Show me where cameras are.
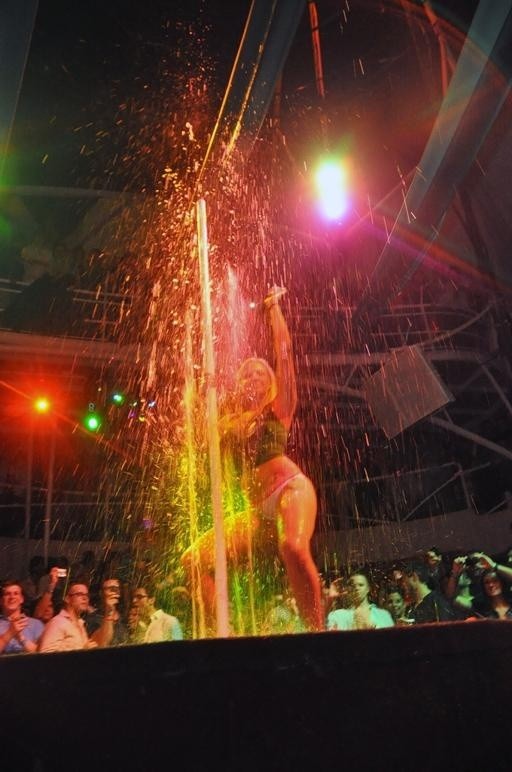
[57,569,67,577]
[335,579,355,592]
[461,556,477,567]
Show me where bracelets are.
[268,302,279,311]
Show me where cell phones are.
[110,585,120,592]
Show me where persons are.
[2,545,511,656]
[180,285,327,639]
